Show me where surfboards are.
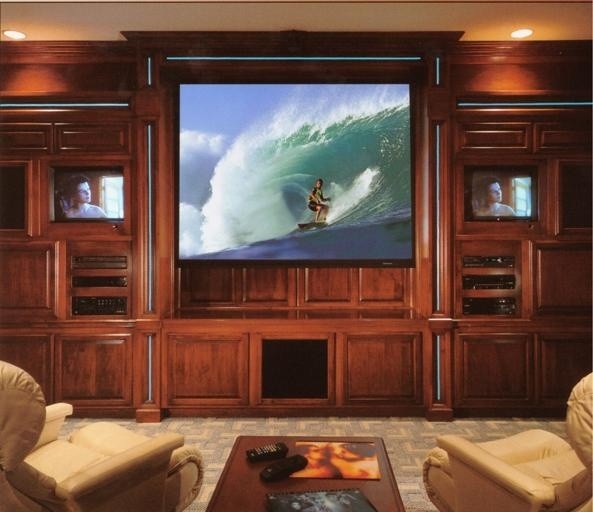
[297,221,328,227]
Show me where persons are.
[308,179,330,222]
[293,444,342,478]
[472,176,516,216]
[290,499,301,511]
[64,175,107,217]
[328,442,379,479]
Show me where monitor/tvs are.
[54,165,126,222]
[464,164,538,222]
[173,78,417,271]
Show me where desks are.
[205,435,406,512]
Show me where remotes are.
[246,442,288,462]
[260,455,308,482]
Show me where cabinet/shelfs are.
[161,319,437,419]
[2,39,145,321]
[454,40,593,319]
[1,327,150,418]
[440,325,593,419]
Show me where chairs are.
[423,373,593,512]
[0,360,205,512]
